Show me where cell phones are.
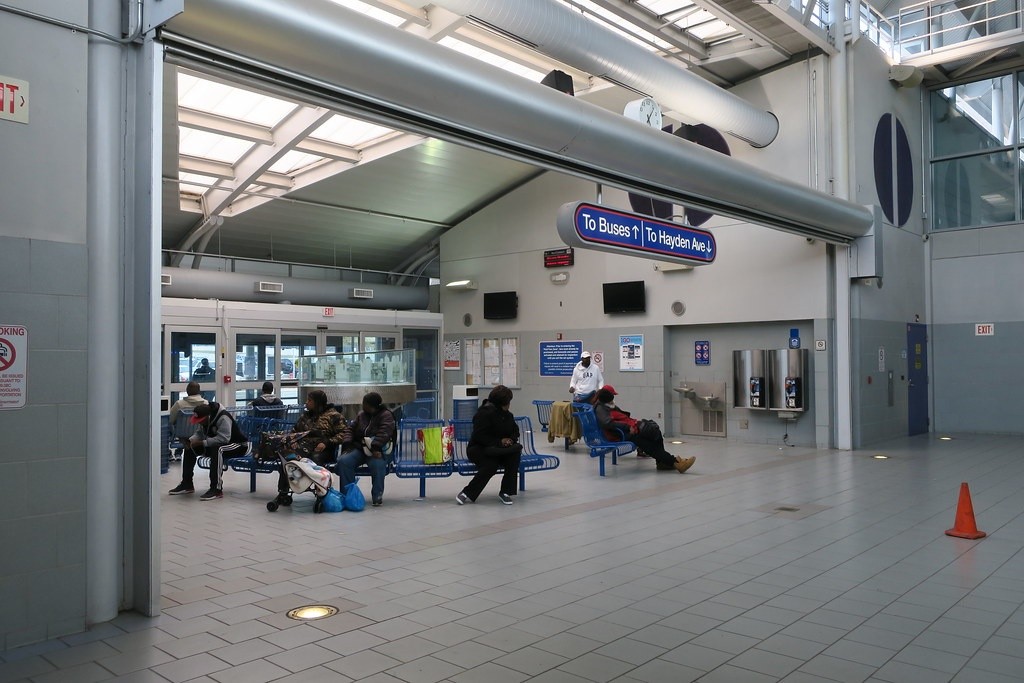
[177,435,189,442]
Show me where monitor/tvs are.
[484,292,517,319]
[602,280,646,313]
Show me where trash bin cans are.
[453,384,478,441]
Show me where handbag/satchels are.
[322,487,345,512]
[417,426,453,464]
[362,437,393,456]
[344,477,365,511]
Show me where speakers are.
[540,69,574,96]
[672,126,702,145]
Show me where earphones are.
[209,416,210,419]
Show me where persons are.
[169,382,210,461]
[456,385,523,505]
[592,385,696,474]
[247,381,283,437]
[569,351,604,445]
[273,390,348,505]
[169,401,248,501]
[190,359,215,403]
[337,392,395,507]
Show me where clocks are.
[624,96,662,131]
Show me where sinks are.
[699,396,720,401]
[672,388,694,392]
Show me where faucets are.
[684,383,688,389]
[710,392,714,397]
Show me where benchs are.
[170,397,635,498]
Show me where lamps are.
[658,260,693,272]
[446,279,478,290]
[888,65,925,90]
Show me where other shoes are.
[200,487,223,500]
[372,492,382,505]
[674,455,696,473]
[273,494,286,503]
[169,480,194,494]
[499,491,513,504]
[636,451,653,459]
[456,491,467,504]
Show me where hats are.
[191,404,211,423]
[581,351,591,358]
[602,385,618,395]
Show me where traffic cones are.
[945,482,986,539]
